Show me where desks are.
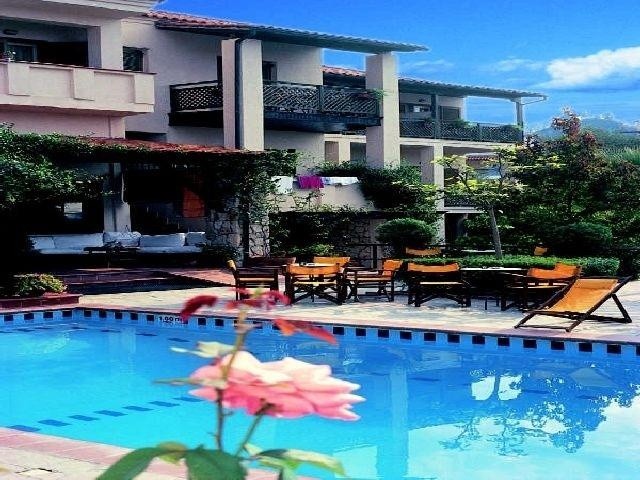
[82,246,140,267]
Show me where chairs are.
[227,252,403,306]
[404,247,582,311]
[514,275,632,332]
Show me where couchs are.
[27,232,206,255]
